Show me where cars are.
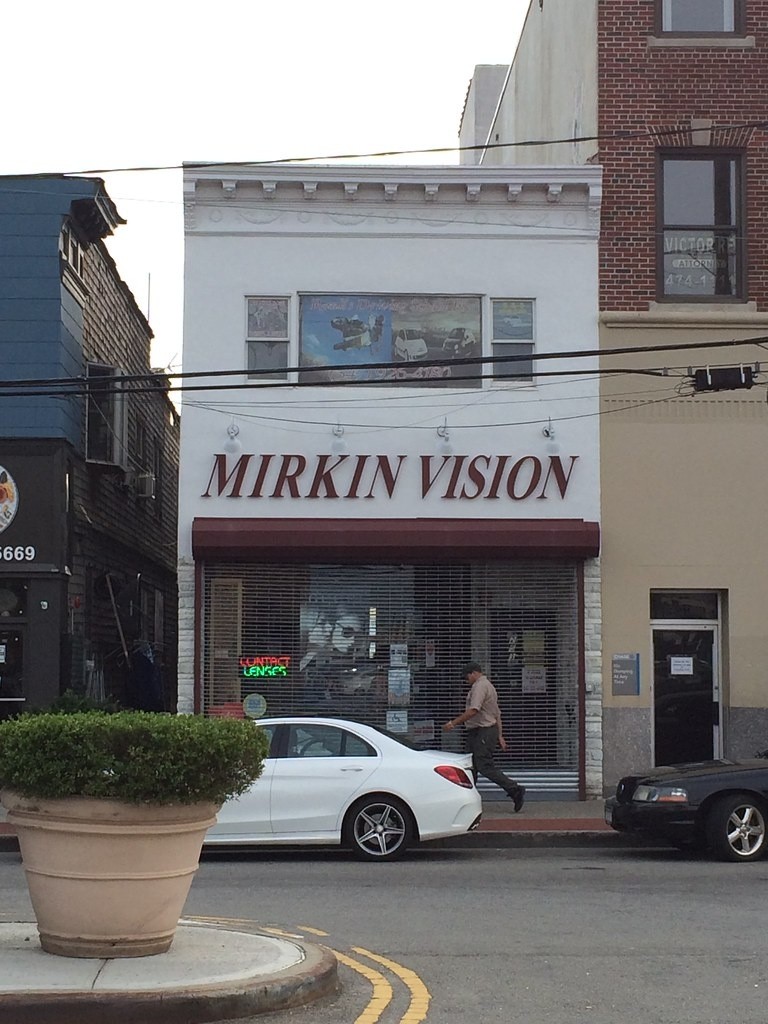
[604,751,768,861]
[200,718,482,861]
[337,664,421,698]
[656,690,718,749]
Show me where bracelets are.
[448,720,455,730]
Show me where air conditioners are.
[135,474,156,497]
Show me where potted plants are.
[0,711,269,958]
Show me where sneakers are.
[506,785,526,812]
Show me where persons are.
[442,661,525,812]
